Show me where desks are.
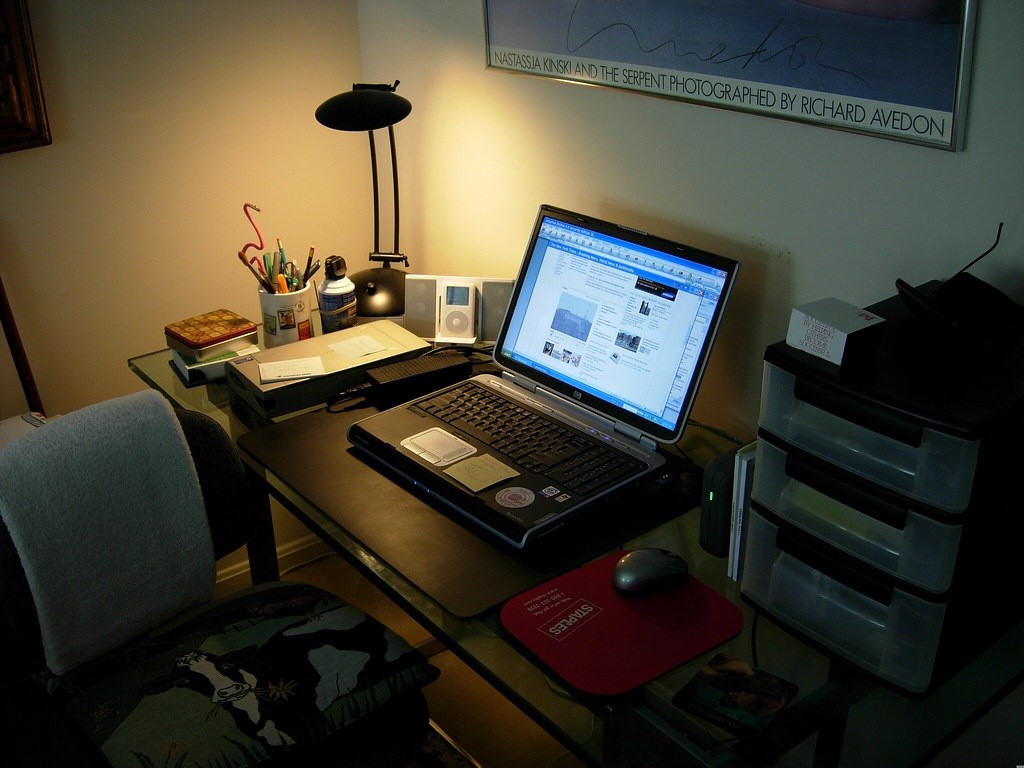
[128,323,1024,768]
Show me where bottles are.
[317,255,357,334]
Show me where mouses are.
[612,547,689,598]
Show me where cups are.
[258,281,314,349]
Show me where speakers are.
[402,272,516,342]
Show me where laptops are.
[345,204,741,551]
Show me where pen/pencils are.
[238,201,323,295]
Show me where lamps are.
[315,80,412,316]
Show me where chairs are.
[0,389,440,768]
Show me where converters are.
[345,349,474,411]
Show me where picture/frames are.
[0,0,52,155]
